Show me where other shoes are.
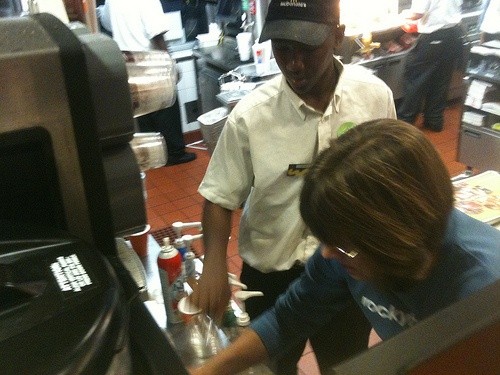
[421,122,443,132]
[167,152,196,164]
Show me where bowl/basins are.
[196,33,220,47]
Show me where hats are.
[258,0,340,46]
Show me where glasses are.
[334,246,360,264]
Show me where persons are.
[188,0,398,375]
[396,0,464,133]
[188,117,500,375]
[99,0,197,167]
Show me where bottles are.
[157,237,185,324]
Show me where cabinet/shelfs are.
[457,38,500,173]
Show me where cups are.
[128,224,151,257]
[177,296,203,325]
[121,49,176,170]
[236,32,252,61]
